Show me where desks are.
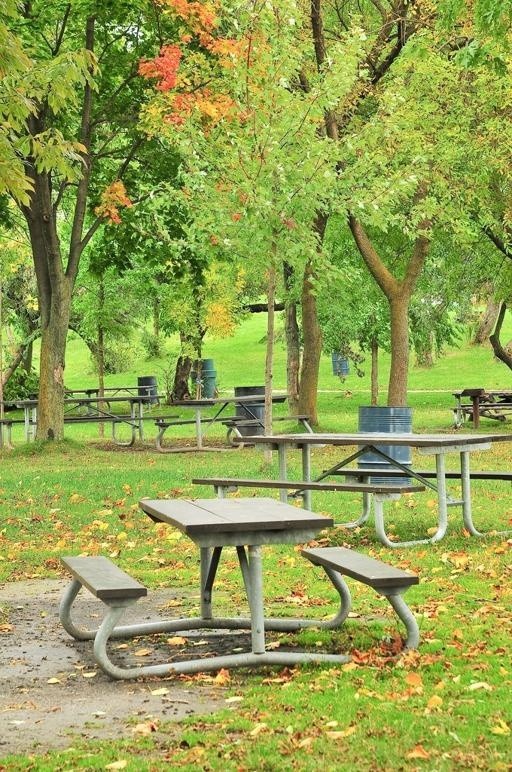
[138,498,335,654]
[1,385,288,452]
[235,433,511,547]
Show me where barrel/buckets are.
[137,376,160,405]
[331,351,351,376]
[191,370,217,399]
[233,386,265,438]
[358,405,413,486]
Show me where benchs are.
[193,469,512,547]
[222,415,315,452]
[298,546,420,668]
[53,555,147,681]
